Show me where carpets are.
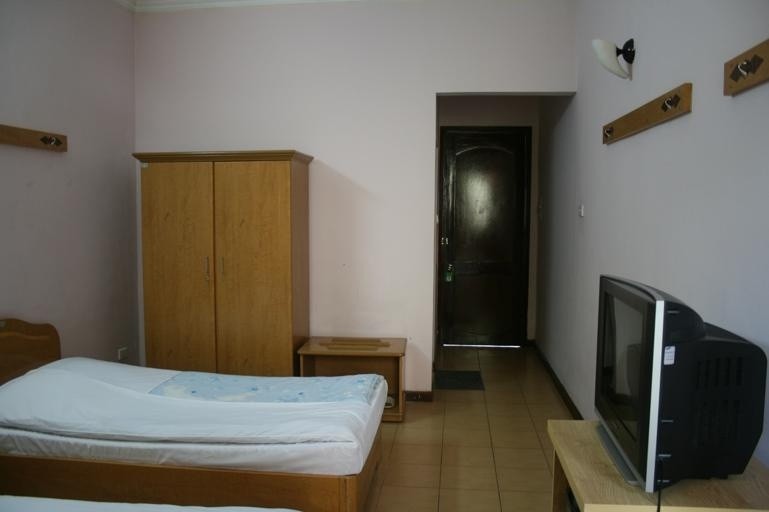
[432,368,485,392]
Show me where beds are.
[2,316,390,511]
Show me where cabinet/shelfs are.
[297,334,408,426]
[132,147,313,375]
[545,419,769,512]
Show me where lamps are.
[589,36,636,79]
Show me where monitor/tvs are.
[593,274,767,496]
